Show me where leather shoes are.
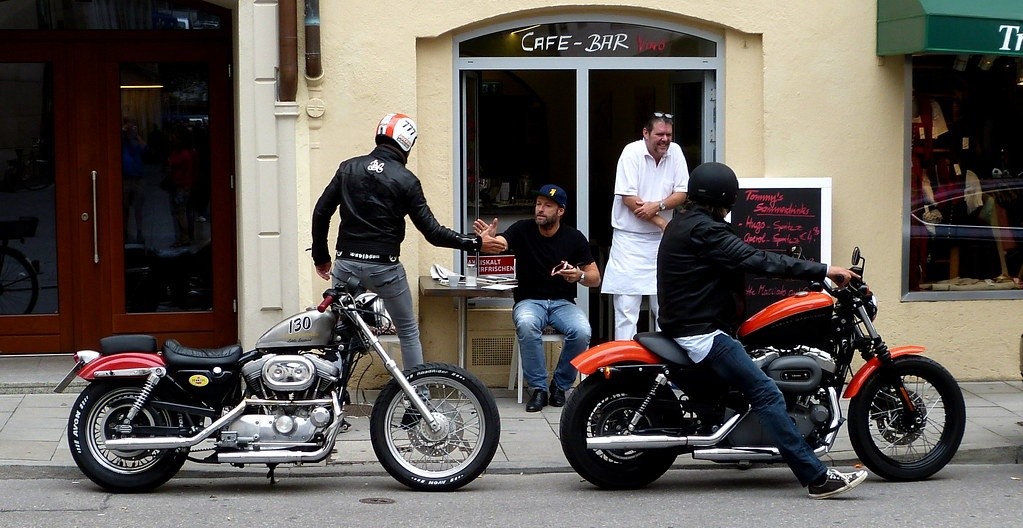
[526,389,548,412]
[548,379,566,407]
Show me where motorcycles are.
[54,272,501,495]
[559,244,966,490]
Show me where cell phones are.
[551,262,568,276]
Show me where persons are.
[656,162,868,500]
[311,113,505,432]
[473,184,601,412]
[122,117,148,244]
[600,112,690,341]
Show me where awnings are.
[876,0,1023,59]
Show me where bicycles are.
[0,218,44,316]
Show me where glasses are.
[647,112,673,126]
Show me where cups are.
[448,273,459,287]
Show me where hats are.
[530,184,566,208]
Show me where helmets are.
[375,114,418,151]
[688,162,738,210]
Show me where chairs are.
[510,258,589,404]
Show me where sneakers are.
[807,470,867,499]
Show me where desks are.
[418,275,516,402]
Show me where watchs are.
[659,201,665,211]
[579,273,585,283]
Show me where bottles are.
[465,264,478,287]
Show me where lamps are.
[977,55,998,71]
[1015,57,1023,86]
[952,53,972,71]
